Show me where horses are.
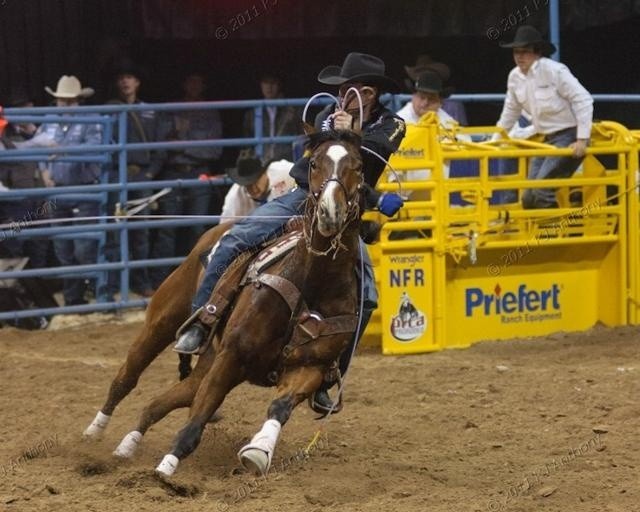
[81,128,364,482]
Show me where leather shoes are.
[174,320,211,357]
[310,388,337,412]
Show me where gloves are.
[377,190,404,219]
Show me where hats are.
[404,73,458,96]
[317,52,401,89]
[45,73,96,98]
[405,56,454,70]
[224,149,277,187]
[497,27,558,56]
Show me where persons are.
[220,154,296,224]
[0,103,66,266]
[387,73,459,183]
[36,75,105,314]
[487,24,593,209]
[174,50,407,413]
[230,66,303,161]
[106,60,157,307]
[404,55,467,126]
[152,61,223,288]
